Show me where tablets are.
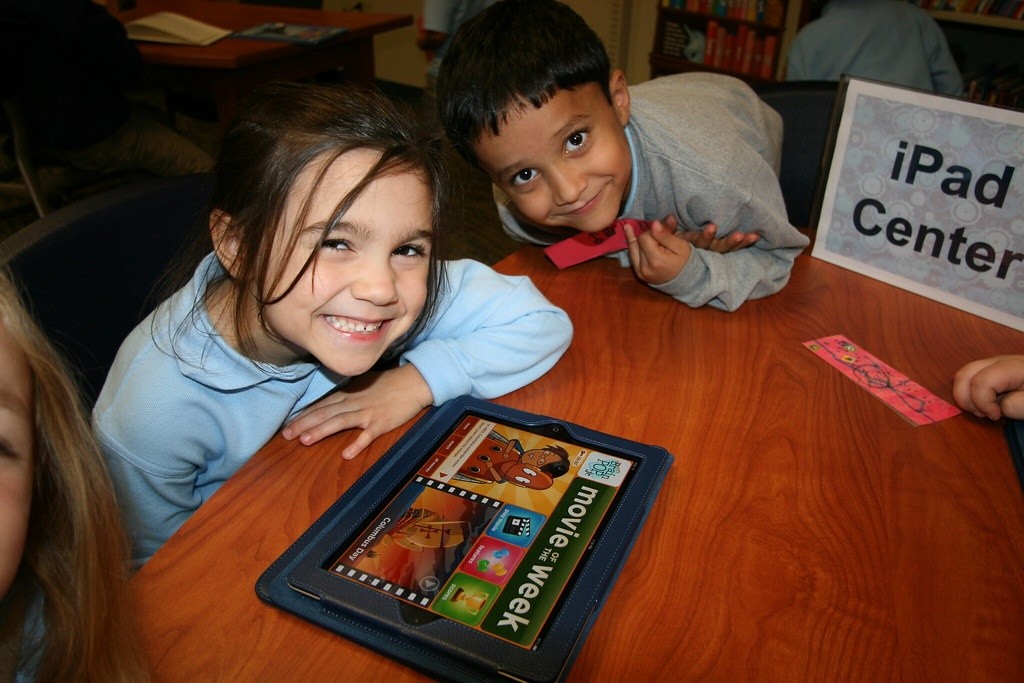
[256,395,676,683]
[227,20,347,47]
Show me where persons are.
[1,0,222,197]
[951,351,1024,418]
[0,271,149,683]
[436,0,809,314]
[779,0,963,104]
[89,63,575,569]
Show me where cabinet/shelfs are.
[649,0,790,99]
[909,0,1024,113]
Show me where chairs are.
[0,93,218,264]
[748,76,849,220]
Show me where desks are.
[126,244,1024,683]
[109,0,414,232]
